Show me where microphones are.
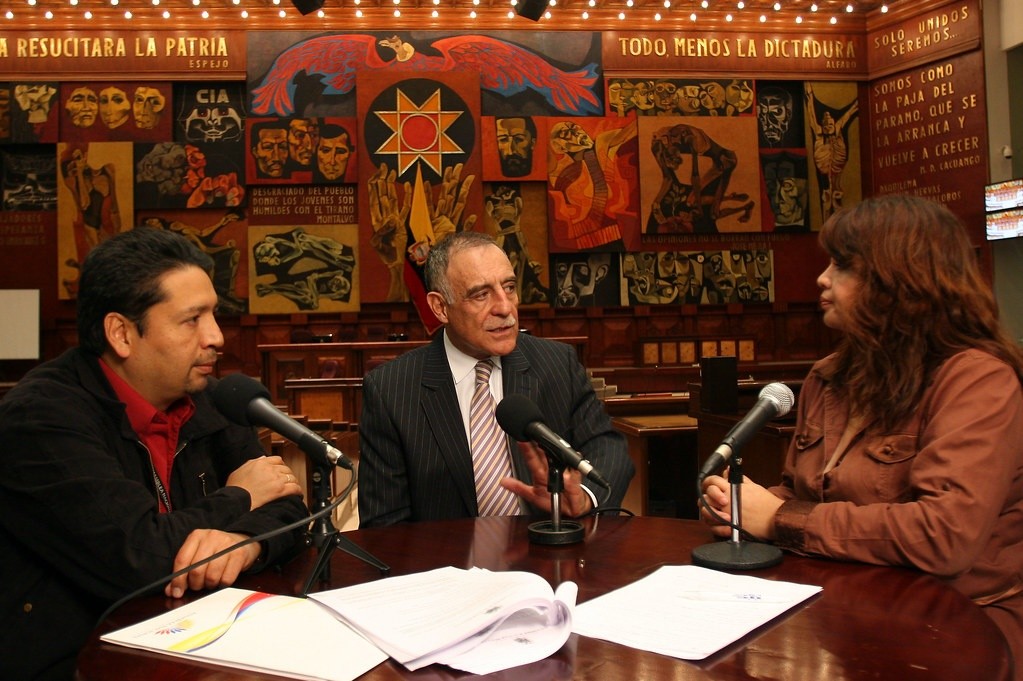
[697,383,795,480]
[495,394,610,490]
[213,373,354,472]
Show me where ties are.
[471,358,522,518]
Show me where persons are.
[693,190,1021,678]
[0,227,316,681]
[353,234,638,530]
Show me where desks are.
[598,379,798,517]
[75,517,1013,681]
[257,335,588,453]
